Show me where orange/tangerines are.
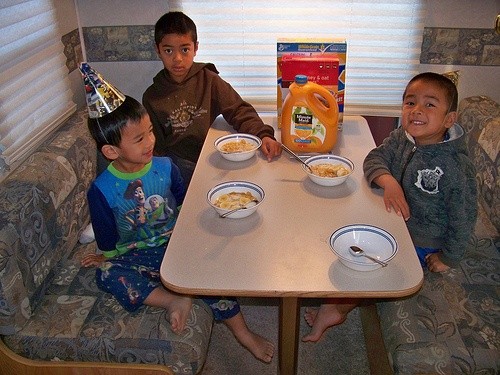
[290,128,322,148]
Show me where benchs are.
[375,96,500,375]
[0,106,212,375]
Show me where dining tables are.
[160,112,424,375]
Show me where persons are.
[81,95,275,363]
[78,12,282,245]
[302,72,479,342]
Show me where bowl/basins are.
[214,133,262,162]
[205,180,266,218]
[327,223,399,272]
[302,154,354,187]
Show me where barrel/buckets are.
[281,74,338,154]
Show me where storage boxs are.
[276,38,346,131]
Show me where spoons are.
[348,246,388,267]
[220,200,259,218]
